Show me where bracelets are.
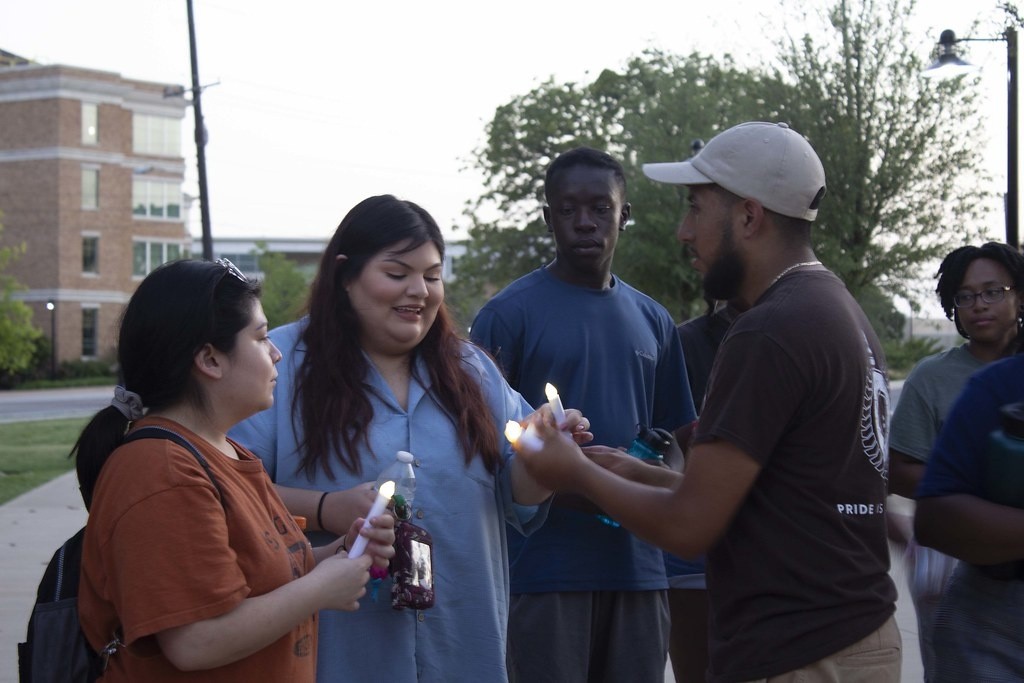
[317,491,329,532]
[334,534,347,552]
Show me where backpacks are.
[18,427,224,683]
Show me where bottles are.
[373,450,418,527]
[595,422,674,528]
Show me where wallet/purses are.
[391,521,435,612]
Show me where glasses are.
[953,286,1013,307]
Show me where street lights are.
[46,299,56,381]
[920,26,1018,247]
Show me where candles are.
[545,382,574,441]
[348,480,396,559]
[504,421,544,453]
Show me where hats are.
[642,120,826,222]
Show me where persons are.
[225,192,593,682]
[668,296,749,682]
[503,120,903,682]
[912,349,1022,682]
[466,148,696,683]
[889,241,1022,682]
[66,256,395,682]
